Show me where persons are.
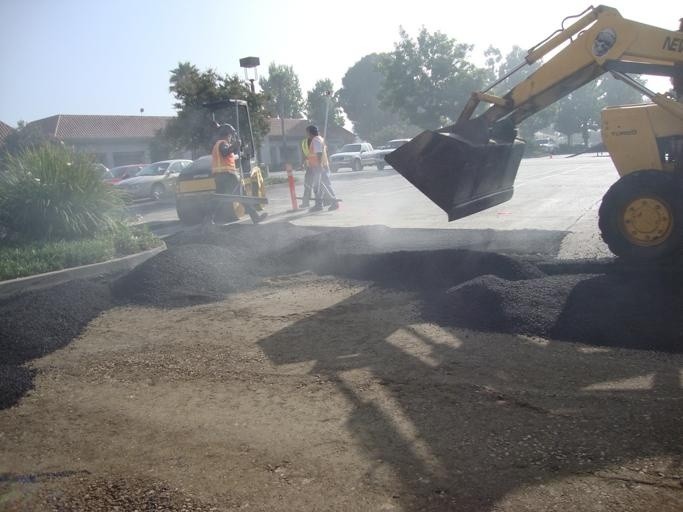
[210,123,269,223]
[305,126,340,212]
[299,136,331,207]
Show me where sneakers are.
[299,203,339,212]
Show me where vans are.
[376,138,412,170]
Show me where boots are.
[250,212,268,223]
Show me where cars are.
[175,151,267,221]
[90,159,193,204]
[535,137,559,154]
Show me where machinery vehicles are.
[383,6,682,263]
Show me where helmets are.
[217,123,235,135]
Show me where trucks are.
[328,142,398,171]
